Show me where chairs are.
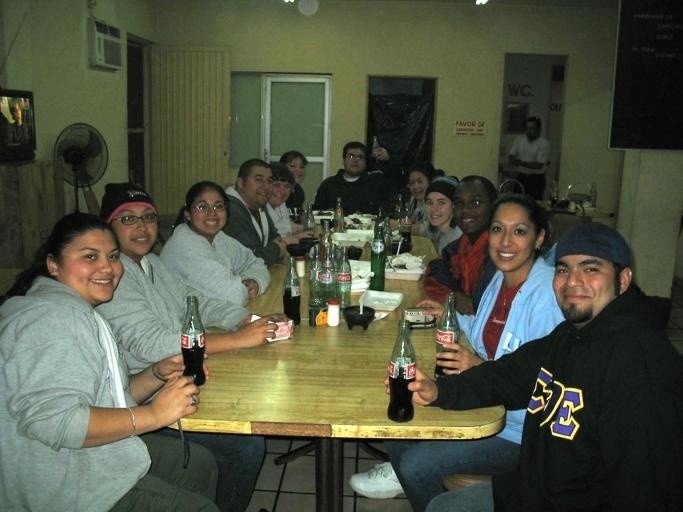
[549,212,592,247]
[499,177,526,198]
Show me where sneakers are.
[349,462,407,499]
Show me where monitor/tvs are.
[0,89,36,163]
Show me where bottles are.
[434,294,460,379]
[387,320,417,423]
[180,295,206,387]
[283,194,413,327]
[372,136,379,156]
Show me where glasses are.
[345,153,362,161]
[190,202,228,212]
[112,212,157,225]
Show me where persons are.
[389,163,462,253]
[510,118,550,201]
[348,176,503,498]
[389,197,570,511]
[96,182,295,512]
[312,141,407,214]
[223,151,307,268]
[385,223,683,510]
[161,181,271,308]
[0,212,220,512]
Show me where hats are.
[423,176,459,205]
[554,222,631,266]
[99,182,159,225]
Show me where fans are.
[52,121,110,218]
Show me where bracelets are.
[151,359,167,385]
[127,406,139,439]
